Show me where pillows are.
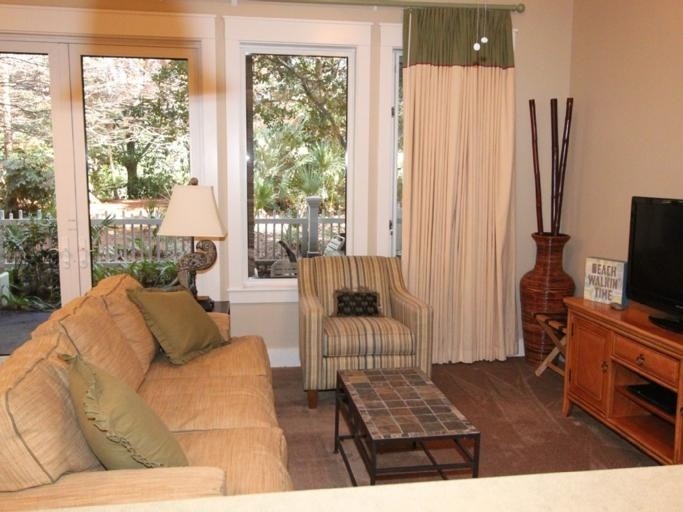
[329,284,386,320]
[122,284,225,366]
[54,353,190,472]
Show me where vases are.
[515,232,575,368]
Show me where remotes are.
[611,302,624,310]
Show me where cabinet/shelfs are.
[560,293,682,466]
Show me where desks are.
[198,300,230,315]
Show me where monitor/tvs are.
[627,197,683,335]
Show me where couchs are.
[289,255,434,410]
[0,270,297,510]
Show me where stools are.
[528,310,569,379]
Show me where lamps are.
[155,175,227,313]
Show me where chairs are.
[249,234,344,275]
